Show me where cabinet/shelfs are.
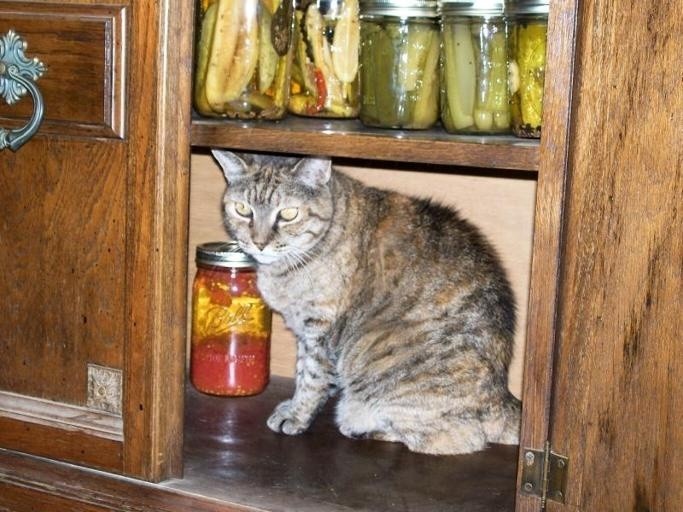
[515,4,683,512]
[0,0,157,510]
[158,1,581,512]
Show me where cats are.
[209,146,522,458]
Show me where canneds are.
[193,0,285,122]
[502,0,550,139]
[440,0,510,136]
[285,0,360,120]
[188,241,272,399]
[357,1,439,132]
